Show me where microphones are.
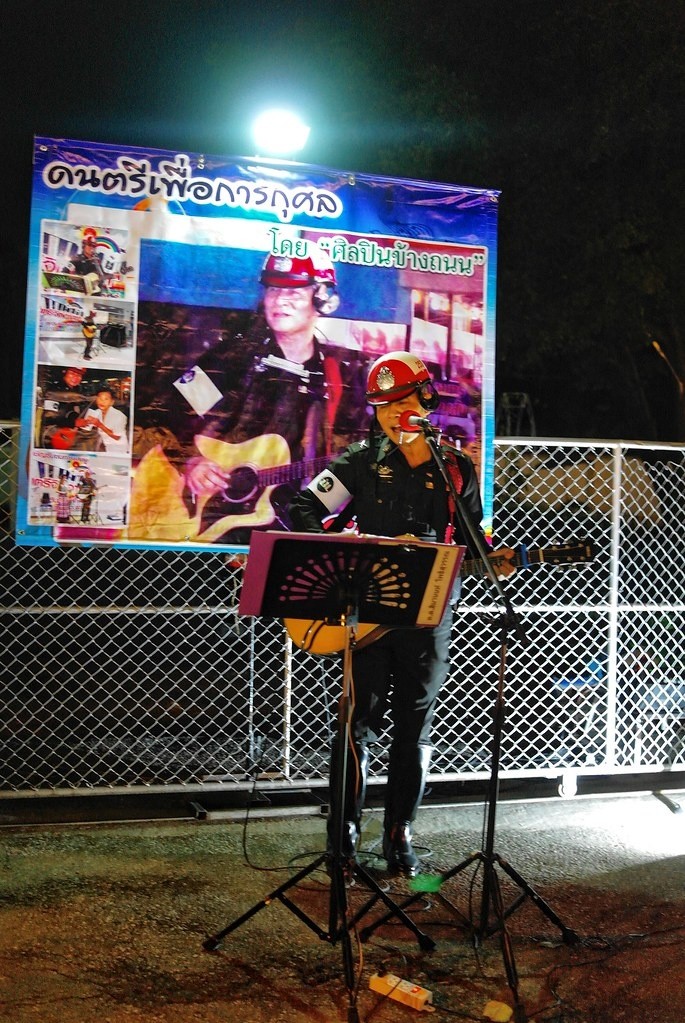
[398,410,432,432]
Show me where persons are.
[138,238,379,546]
[59,235,116,296]
[81,309,102,360]
[285,351,513,889]
[39,366,129,452]
[56,471,98,522]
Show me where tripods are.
[68,489,105,527]
[81,329,110,359]
[357,427,576,1016]
[201,538,438,992]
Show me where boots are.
[383,745,431,877]
[328,736,370,851]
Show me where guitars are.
[284,534,595,657]
[130,434,341,543]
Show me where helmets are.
[258,237,338,288]
[68,367,87,375]
[83,234,97,247]
[366,351,430,405]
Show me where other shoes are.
[84,354,93,361]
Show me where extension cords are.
[369,971,436,1012]
